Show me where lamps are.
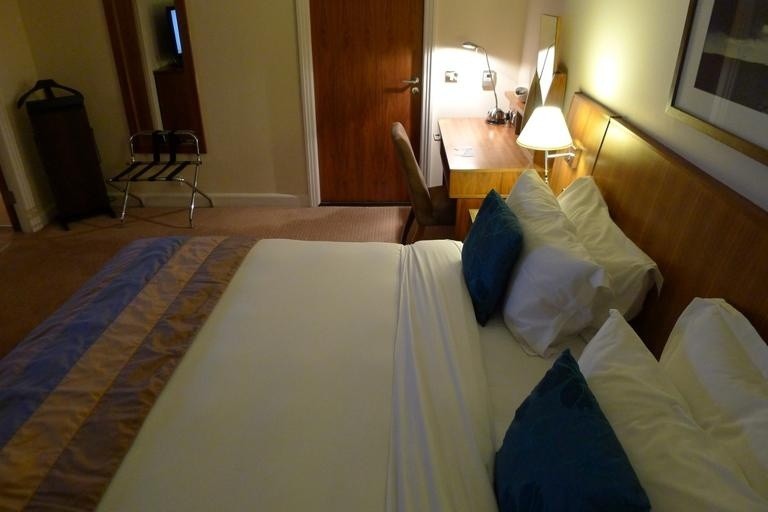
[461,41,504,119]
[516,106,583,184]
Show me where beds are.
[2,93,768,512]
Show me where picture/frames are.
[664,0,767,160]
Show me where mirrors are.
[536,13,560,109]
[102,0,208,155]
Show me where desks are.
[437,114,547,241]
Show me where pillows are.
[494,347,653,511]
[462,189,523,325]
[556,174,664,344]
[578,308,767,511]
[660,296,768,495]
[503,167,610,358]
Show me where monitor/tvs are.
[167,6,183,58]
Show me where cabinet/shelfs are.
[152,64,204,146]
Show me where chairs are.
[106,130,214,228]
[391,121,457,245]
